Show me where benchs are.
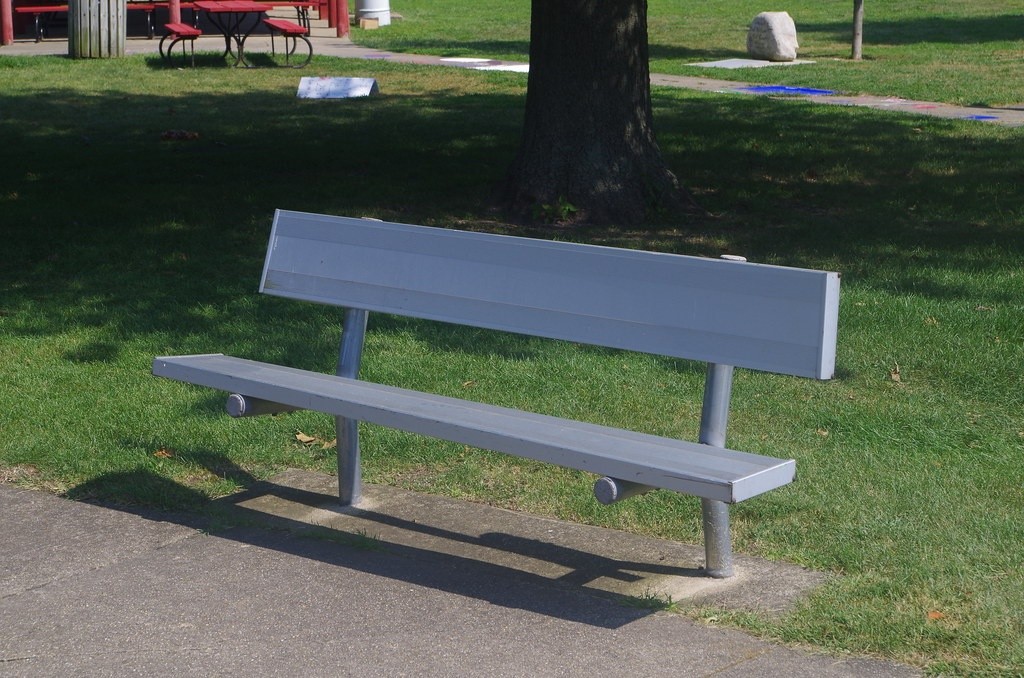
[262,19,314,69]
[157,0,320,38]
[159,23,203,71]
[154,209,842,584]
[14,3,154,44]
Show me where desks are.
[193,1,273,70]
